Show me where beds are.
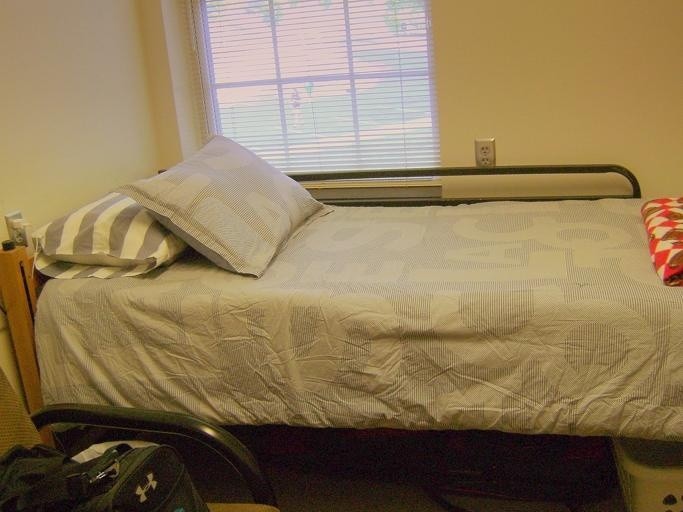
[0,162,683,453]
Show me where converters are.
[13,218,25,230]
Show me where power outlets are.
[4,210,29,248]
[473,136,497,168]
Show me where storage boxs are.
[611,437,683,512]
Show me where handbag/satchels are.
[14,440,210,512]
